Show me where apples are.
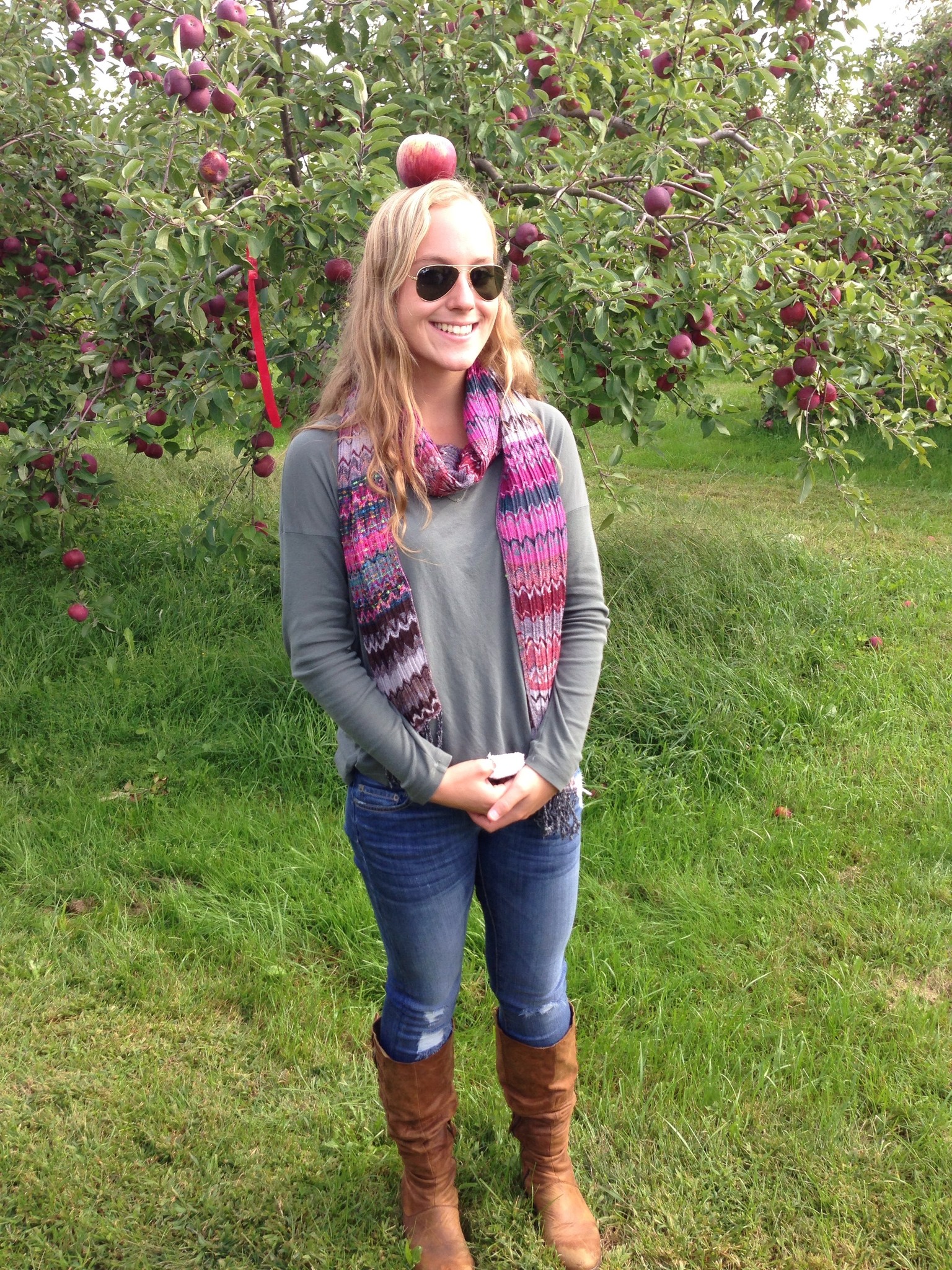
[900,595,918,612]
[587,780,607,798]
[62,597,92,619]
[772,804,792,822]
[1,1,952,572]
[863,632,883,653]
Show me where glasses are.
[407,264,505,302]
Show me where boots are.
[494,1000,601,1270]
[370,1013,475,1270]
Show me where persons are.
[281,177,609,1269]
